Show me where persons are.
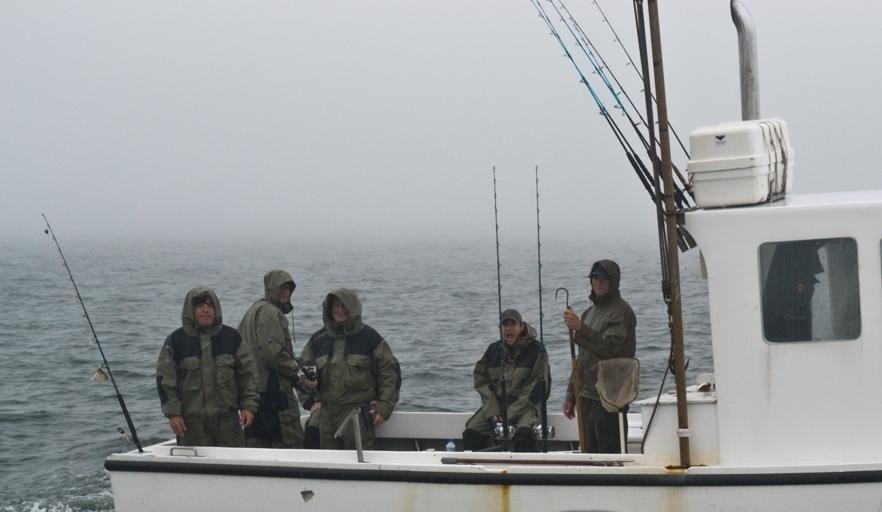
[295,287,401,449]
[462,308,551,451]
[236,269,306,449]
[155,286,261,447]
[562,260,639,453]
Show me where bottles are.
[446,438,456,450]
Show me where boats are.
[40,0,882,512]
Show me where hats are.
[588,266,610,278]
[500,309,520,323]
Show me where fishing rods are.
[492,165,509,452]
[533,1,697,253]
[40,212,144,453]
[267,334,318,381]
[535,166,549,451]
